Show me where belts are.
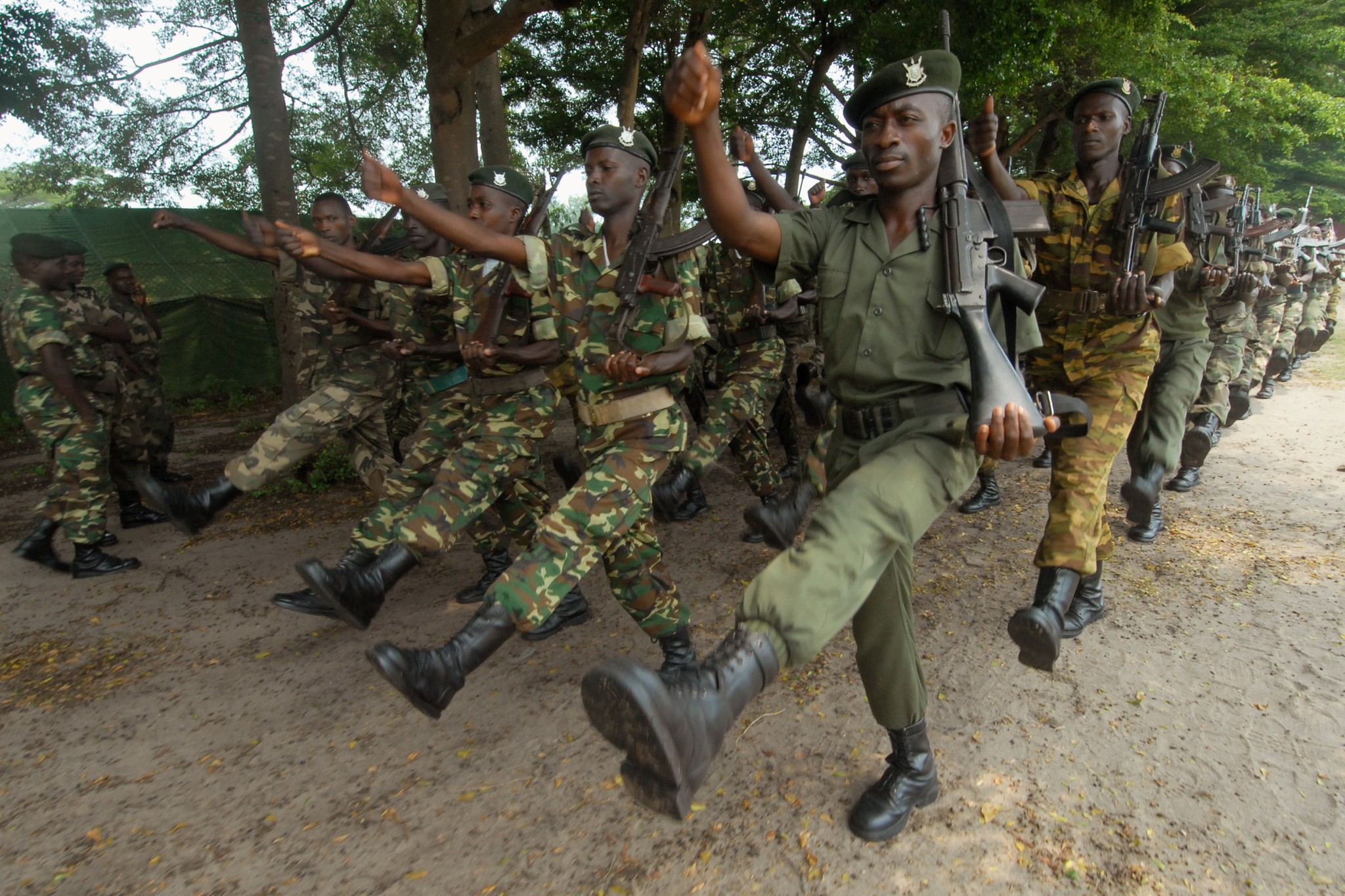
[1039,288,1114,314]
[319,316,971,440]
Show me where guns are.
[611,147,718,366]
[1185,140,1238,273]
[737,272,769,318]
[327,204,414,306]
[1330,237,1345,278]
[1232,182,1284,273]
[1253,186,1292,268]
[1109,92,1221,300]
[1314,238,1329,278]
[471,168,567,346]
[1005,155,1014,176]
[1287,186,1316,282]
[917,8,1051,436]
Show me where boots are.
[364,602,517,718]
[456,548,514,603]
[521,583,591,641]
[15,466,244,577]
[581,630,779,823]
[848,721,939,839]
[655,631,698,673]
[271,543,378,620]
[549,363,834,551]
[958,317,1337,674]
[296,545,419,630]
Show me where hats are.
[60,238,87,255]
[843,50,961,132]
[102,262,129,275]
[580,124,657,172]
[1064,78,1141,121]
[1153,144,1332,233]
[841,152,868,170]
[410,183,448,203]
[741,180,766,205]
[10,233,63,258]
[468,165,534,209]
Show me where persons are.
[151,165,590,644]
[577,78,1342,672]
[0,232,195,581]
[357,125,699,723]
[580,40,1061,842]
